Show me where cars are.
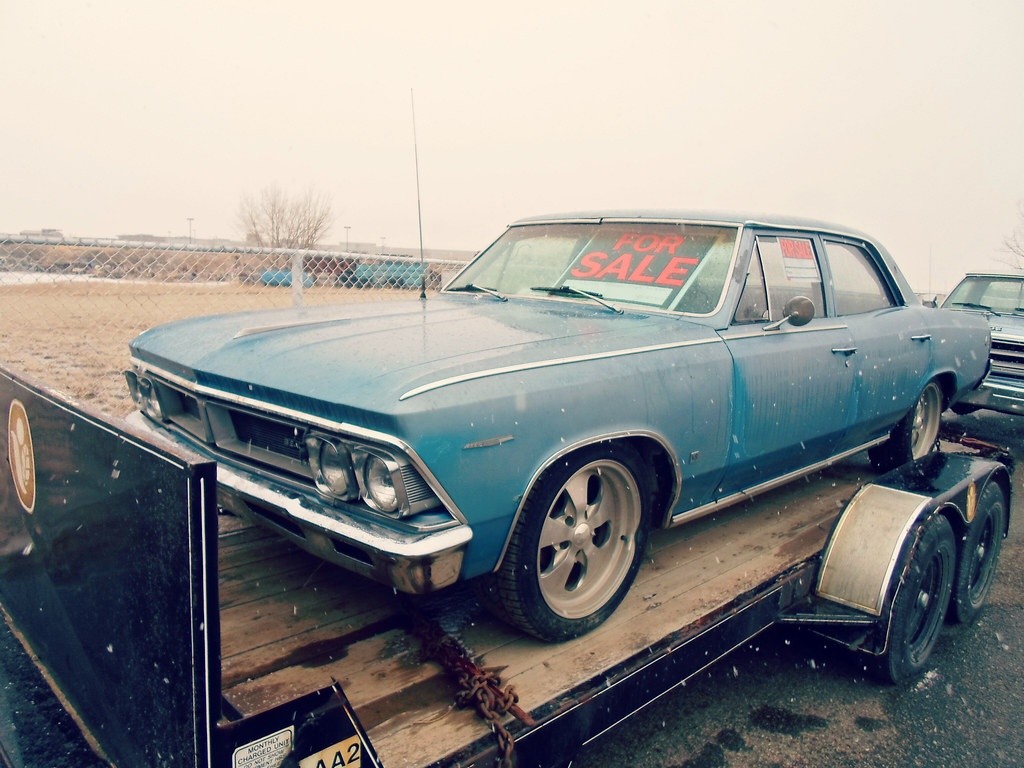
[355,253,441,289]
[261,269,314,287]
[122,207,994,643]
[922,272,1024,416]
